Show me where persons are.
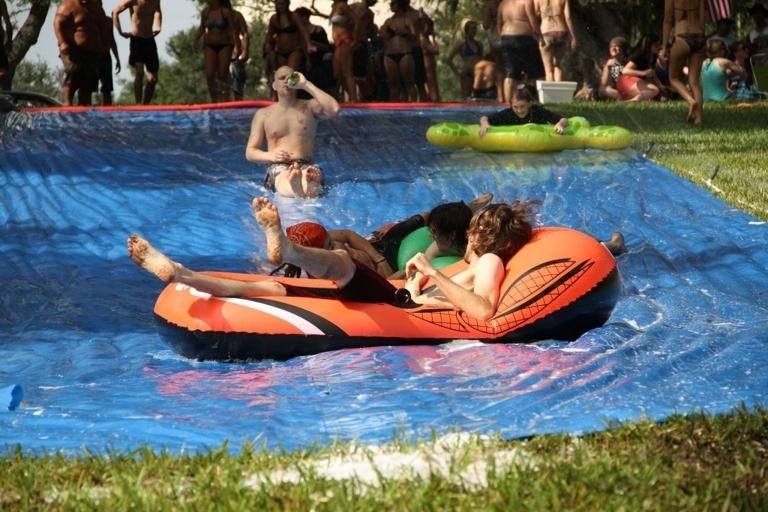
[124,195,537,322]
[384,201,473,281]
[476,83,569,139]
[446,1,766,124]
[190,0,442,106]
[245,65,340,199]
[284,192,494,281]
[112,0,163,105]
[53,0,121,106]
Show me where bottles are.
[288,73,298,86]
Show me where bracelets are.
[376,259,386,264]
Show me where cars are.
[0,90,59,114]
